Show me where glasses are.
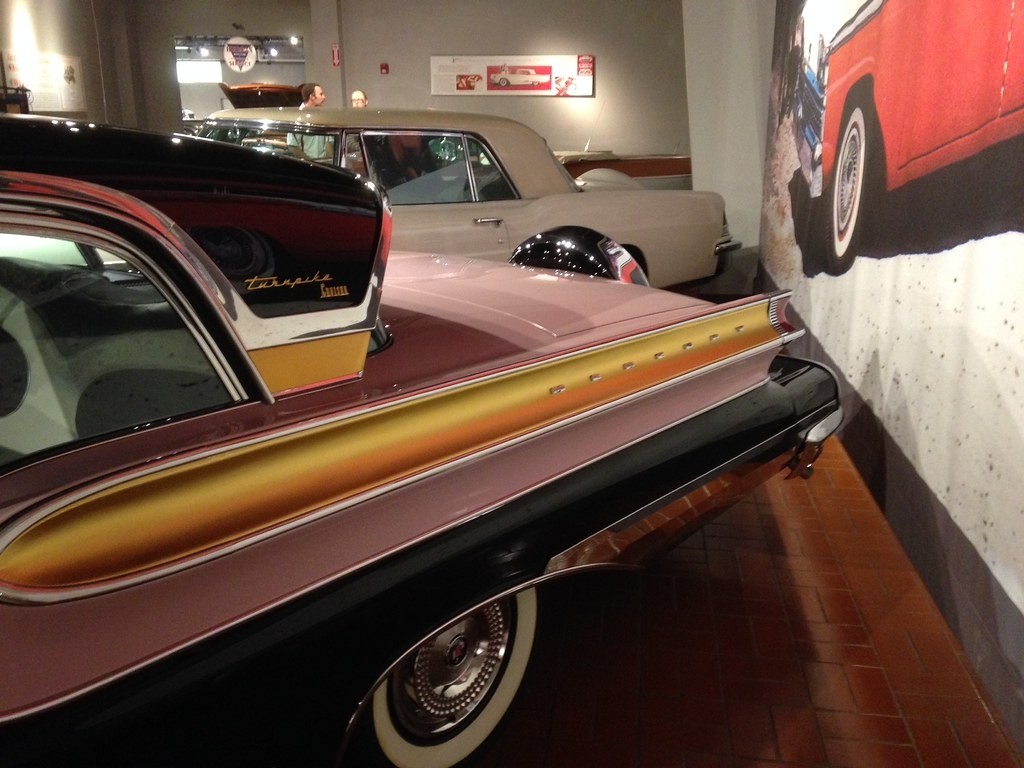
[350,98,364,102]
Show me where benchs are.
[0,254,232,471]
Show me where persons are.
[346,90,368,153]
[287,81,333,163]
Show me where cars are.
[490,67,550,86]
[0,108,846,768]
[193,106,743,295]
[218,81,307,150]
